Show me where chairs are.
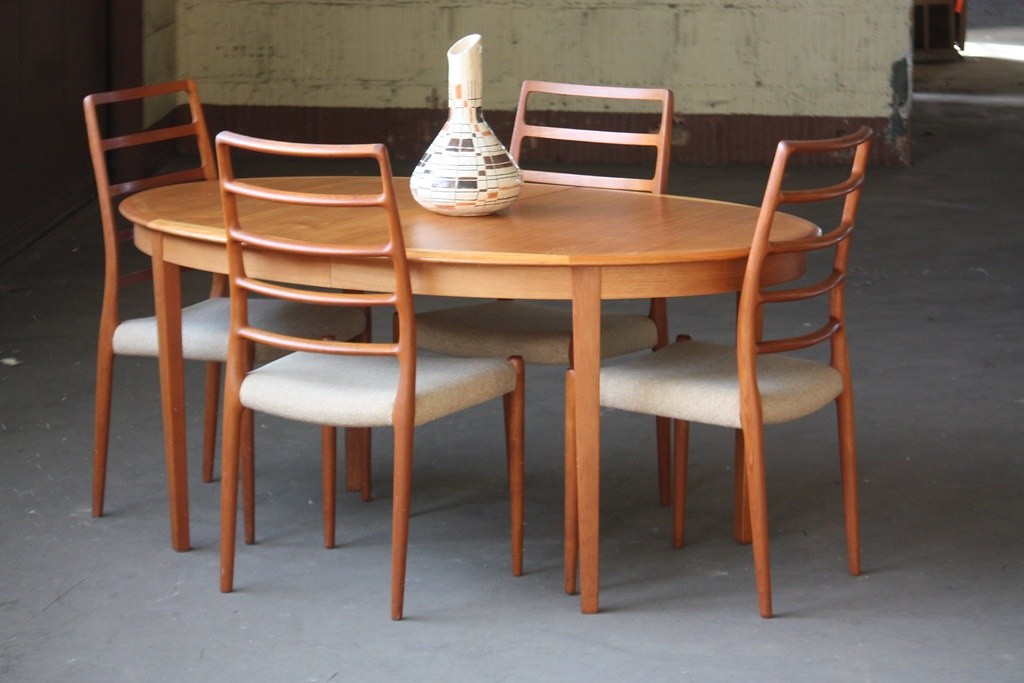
[393,79,675,563]
[81,80,364,547]
[215,130,527,621]
[564,125,874,617]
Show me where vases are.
[408,32,522,220]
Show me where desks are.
[115,173,821,614]
